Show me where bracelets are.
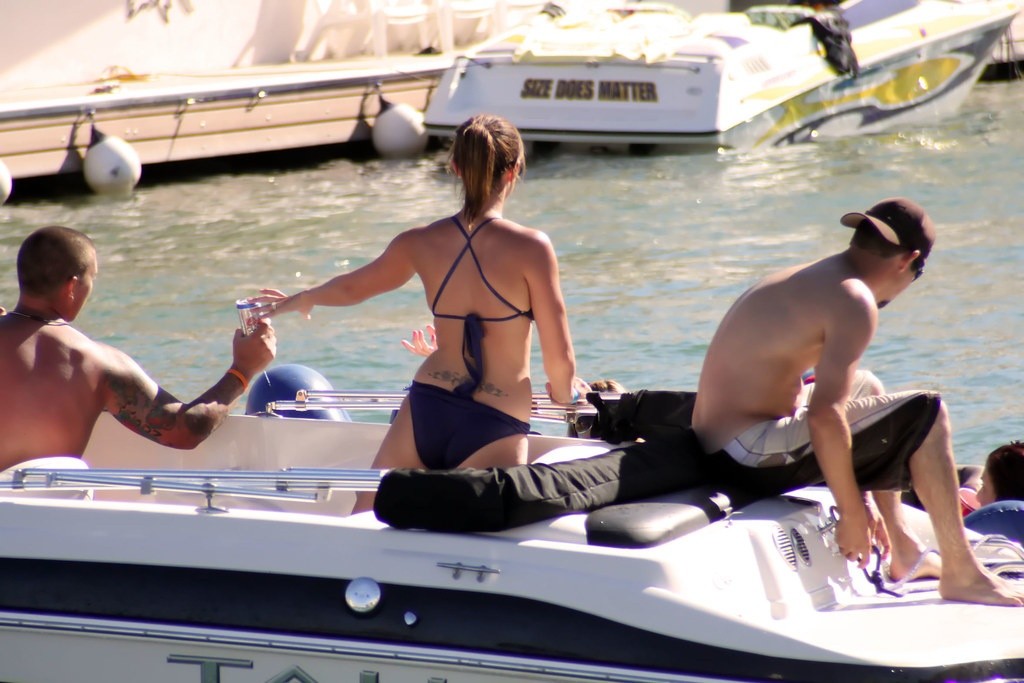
[570,387,578,405]
[225,368,248,392]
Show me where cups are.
[958,487,982,517]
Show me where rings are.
[270,301,276,312]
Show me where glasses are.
[913,261,924,281]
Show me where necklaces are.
[6,309,69,326]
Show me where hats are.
[840,197,936,259]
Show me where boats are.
[1,51,462,211]
[1,388,1024,683]
[418,0,1023,160]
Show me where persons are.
[690,199,1024,607]
[247,115,601,517]
[0,225,277,474]
[901,440,1024,518]
[400,305,628,438]
